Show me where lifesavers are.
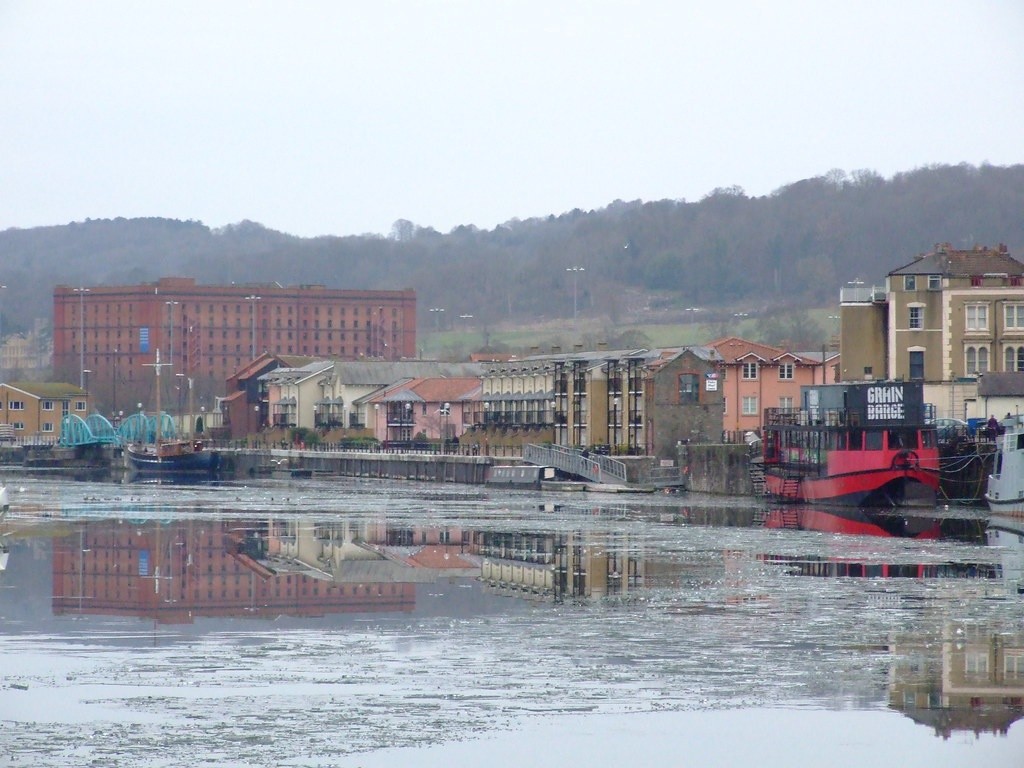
[185,446,191,453]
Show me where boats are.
[745,381,1024,504]
[115,434,220,470]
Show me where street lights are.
[429,307,444,359]
[73,286,90,390]
[565,265,585,319]
[244,295,262,362]
[136,398,620,455]
[165,299,179,376]
